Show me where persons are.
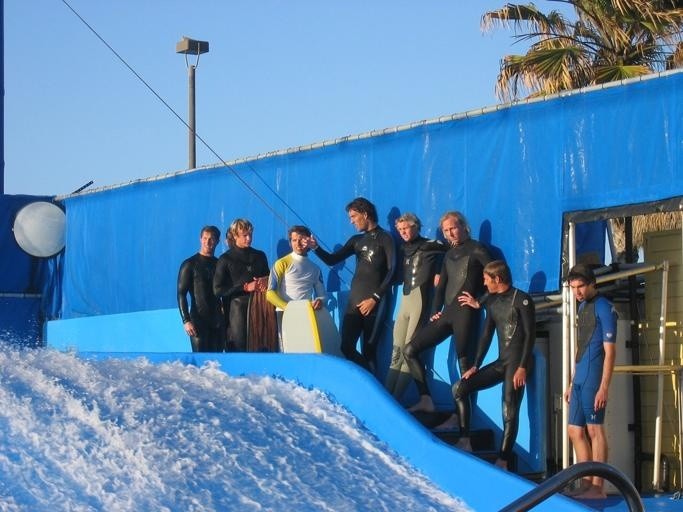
[175,224,225,353]
[397,209,492,433]
[449,258,535,472]
[264,224,327,355]
[300,193,399,379]
[554,262,620,503]
[383,212,444,404]
[212,216,271,353]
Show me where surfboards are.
[282,300,345,359]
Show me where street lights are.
[175,36,209,170]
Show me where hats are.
[569,264,597,280]
